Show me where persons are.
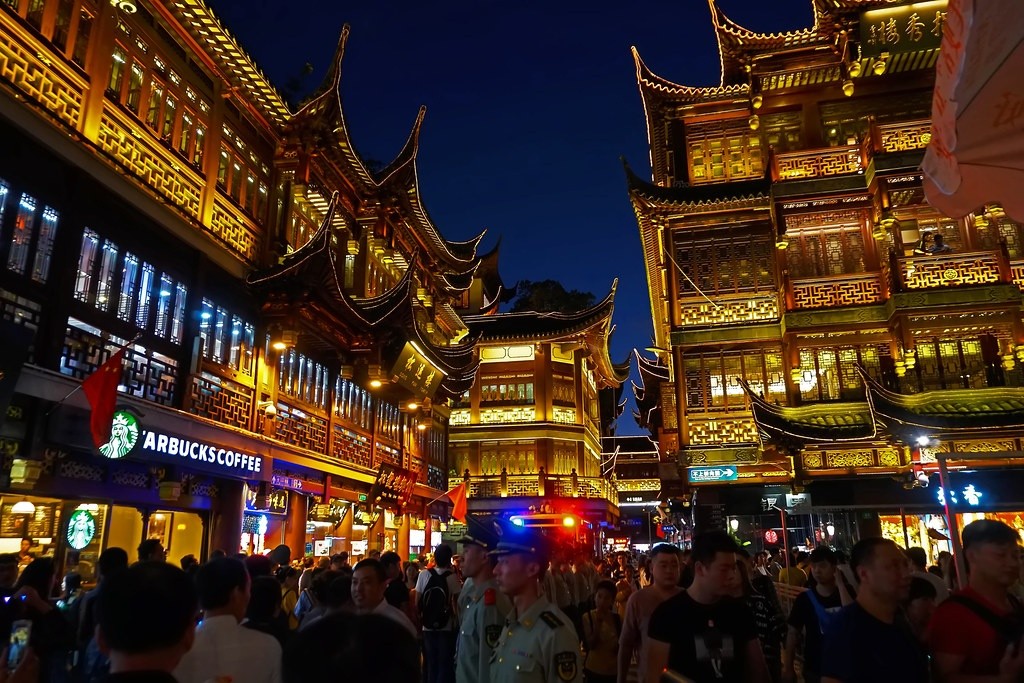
[0,519,1024,683]
[913,232,955,287]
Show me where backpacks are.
[418,568,454,629]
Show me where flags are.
[445,483,467,524]
[81,350,122,448]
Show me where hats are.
[485,517,557,561]
[454,515,500,551]
